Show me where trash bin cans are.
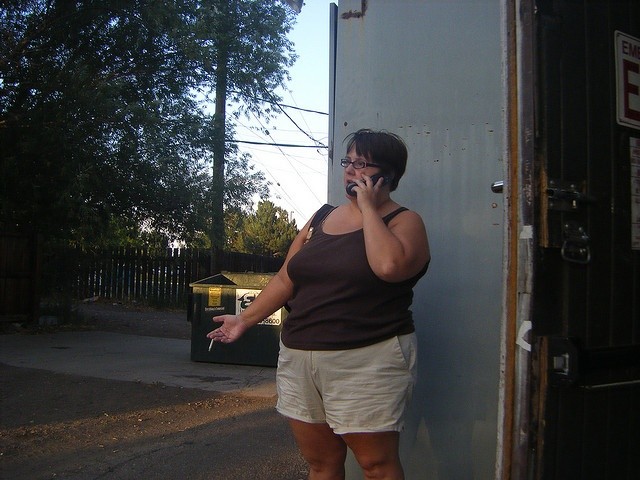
[187,271,288,366]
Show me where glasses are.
[340,158,378,169]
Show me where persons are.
[204,128,431,480]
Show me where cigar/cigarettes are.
[208,339,214,351]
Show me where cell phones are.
[345,173,388,196]
